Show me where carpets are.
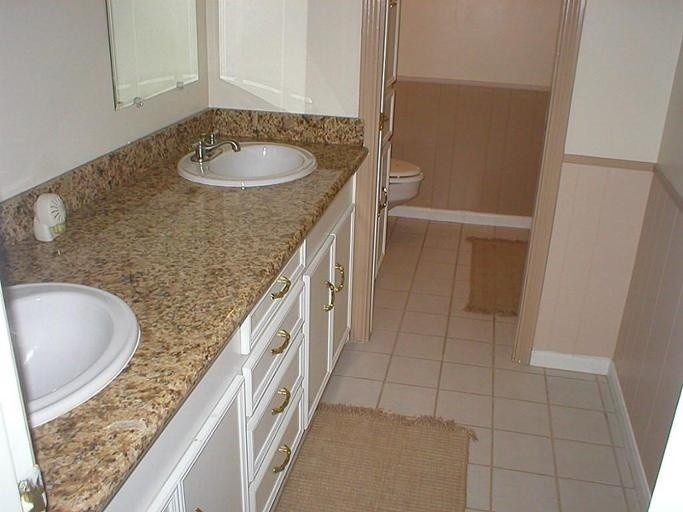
[273,403,477,512]
[463,237,527,316]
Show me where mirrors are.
[106,0,201,112]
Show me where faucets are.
[195,137,241,159]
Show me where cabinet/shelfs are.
[304,175,356,430]
[242,243,302,511]
[105,326,240,511]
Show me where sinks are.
[1,282,141,432]
[176,141,317,188]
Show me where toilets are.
[386,156,425,211]
